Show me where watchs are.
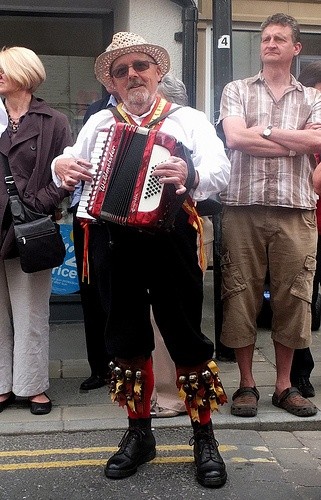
[263,125,273,139]
[289,149,297,156]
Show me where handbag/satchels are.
[7,200,66,273]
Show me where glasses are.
[110,60,158,78]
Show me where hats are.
[93,31,171,93]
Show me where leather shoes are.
[290,370,315,397]
[80,374,110,389]
[105,414,156,478]
[188,418,227,487]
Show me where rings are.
[76,159,80,164]
[173,156,177,161]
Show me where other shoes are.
[0,393,16,413]
[27,391,52,414]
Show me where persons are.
[0,46,75,415]
[50,32,231,487]
[214,11,321,417]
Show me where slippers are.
[271,387,317,417]
[230,385,259,416]
